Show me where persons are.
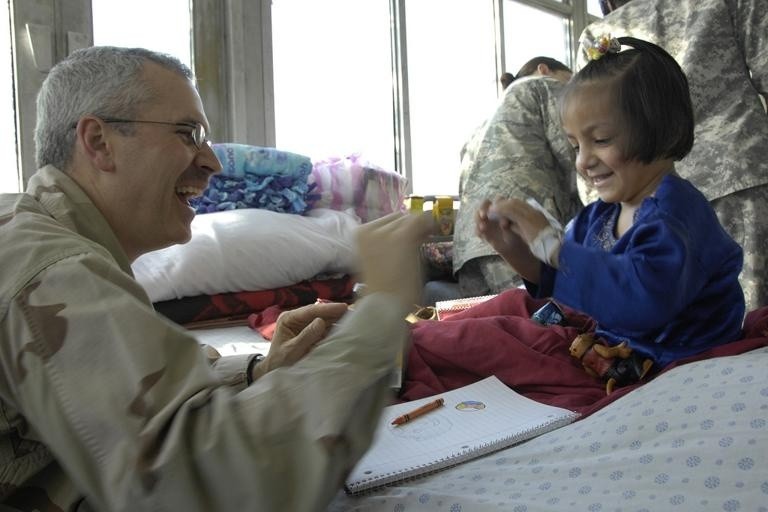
[476,35,745,366]
[0,46,441,511]
[566,331,654,400]
[452,56,584,297]
[574,0,767,329]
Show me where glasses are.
[72,112,211,151]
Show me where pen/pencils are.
[391,398,444,424]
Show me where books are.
[342,373,583,497]
